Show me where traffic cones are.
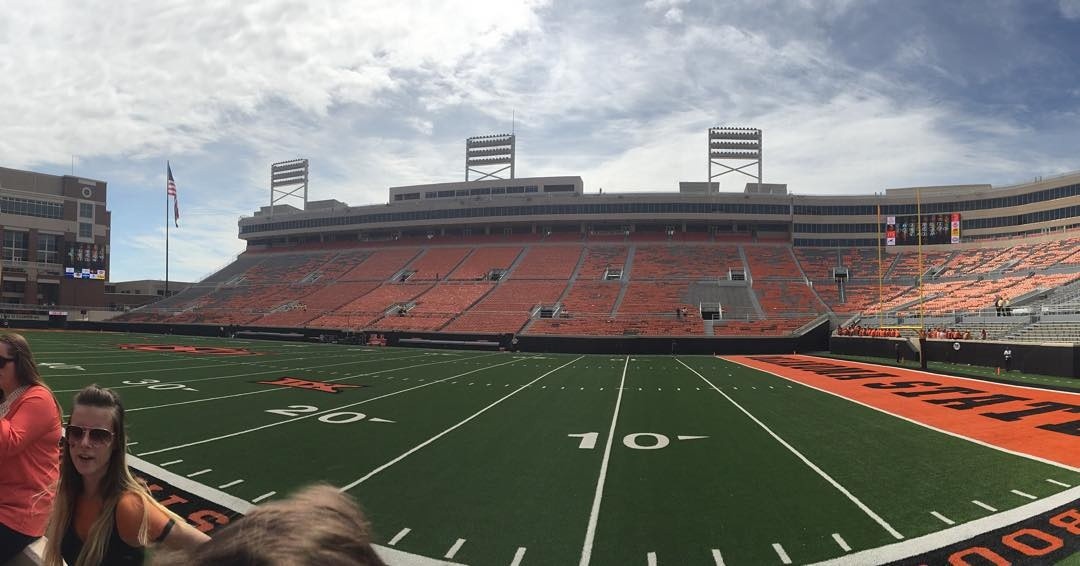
[995,367,1001,375]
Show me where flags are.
[168,165,180,228]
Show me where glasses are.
[0,356,17,369]
[65,425,116,447]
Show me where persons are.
[398,307,406,317]
[894,341,901,363]
[676,307,681,318]
[0,333,62,566]
[682,306,688,317]
[1004,346,1012,373]
[402,273,405,281]
[995,297,1014,316]
[28,384,213,566]
[161,480,388,566]
[836,324,902,338]
[918,327,987,340]
